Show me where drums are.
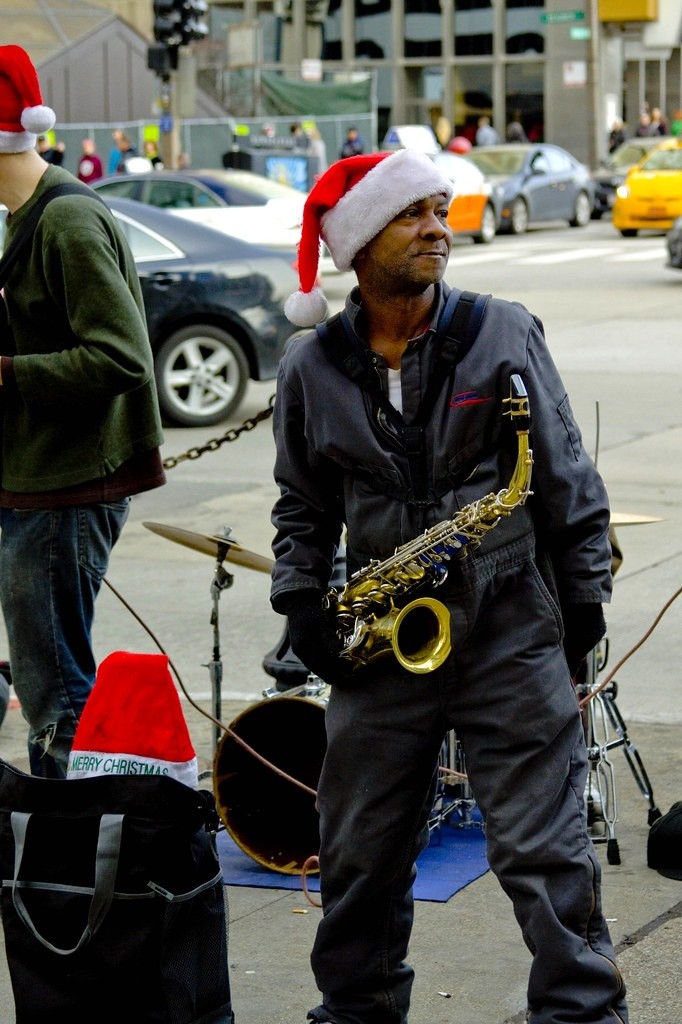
[213,678,446,876]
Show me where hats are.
[284,147,453,328]
[0,44,56,154]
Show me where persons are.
[340,128,364,159]
[144,140,164,170]
[608,120,626,152]
[476,116,498,147]
[0,44,167,778]
[269,149,628,1024]
[430,106,452,146]
[635,107,665,137]
[506,109,526,142]
[108,128,137,175]
[178,152,193,169]
[291,123,327,173]
[36,136,65,166]
[78,138,102,183]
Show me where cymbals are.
[610,511,661,528]
[142,520,276,574]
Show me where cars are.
[0,166,327,428]
[590,136,682,237]
[381,124,595,244]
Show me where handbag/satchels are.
[0,758,235,1024]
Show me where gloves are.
[286,597,365,692]
[561,602,606,678]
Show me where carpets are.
[217,796,490,902]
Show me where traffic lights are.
[153,0,206,44]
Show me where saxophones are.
[324,374,535,674]
